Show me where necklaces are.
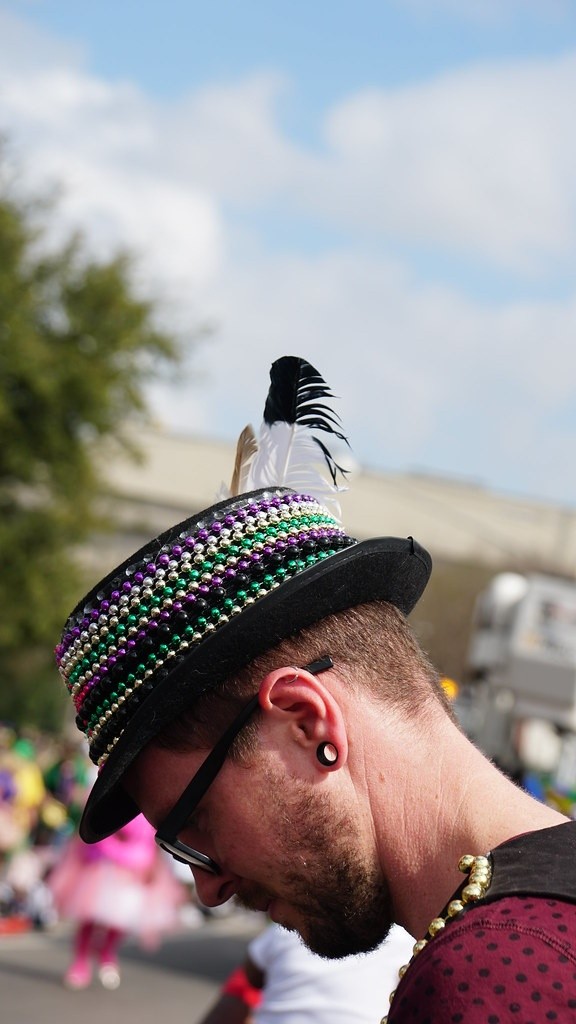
[380,855,492,1024]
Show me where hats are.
[54,356,432,845]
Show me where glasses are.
[154,655,334,878]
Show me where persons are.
[1,486,576,1024]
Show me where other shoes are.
[65,963,91,990]
[98,962,120,990]
